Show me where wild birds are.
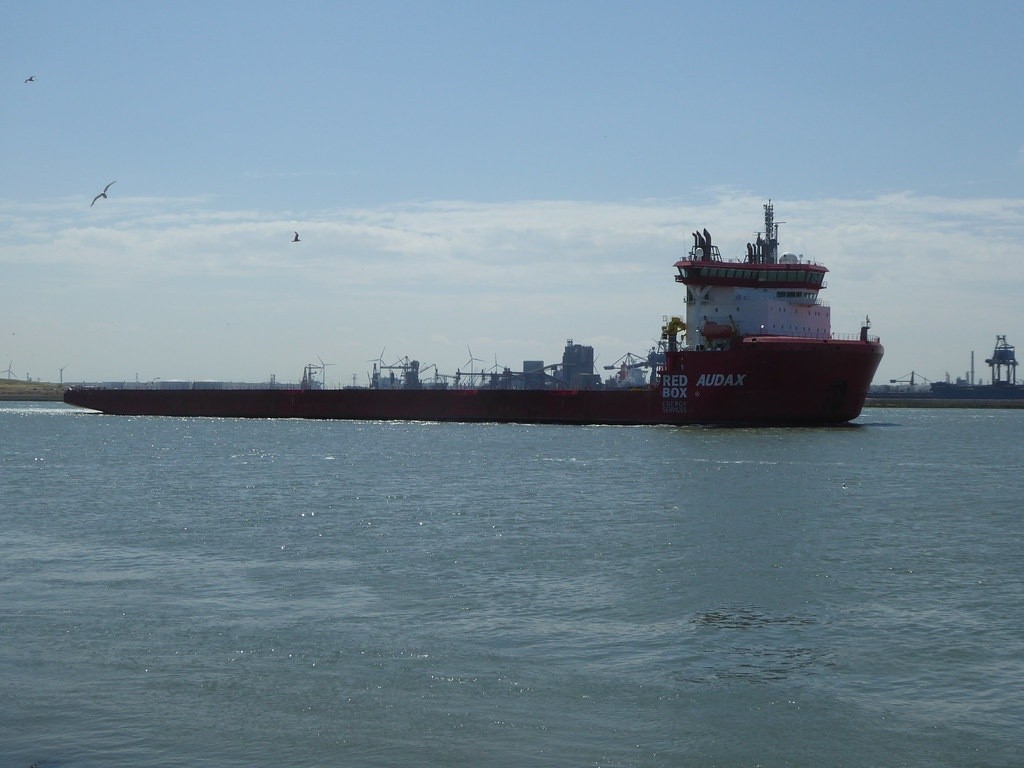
[25,75,36,83]
[89,180,118,208]
[291,231,301,242]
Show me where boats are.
[61,199,887,427]
[703,322,733,341]
